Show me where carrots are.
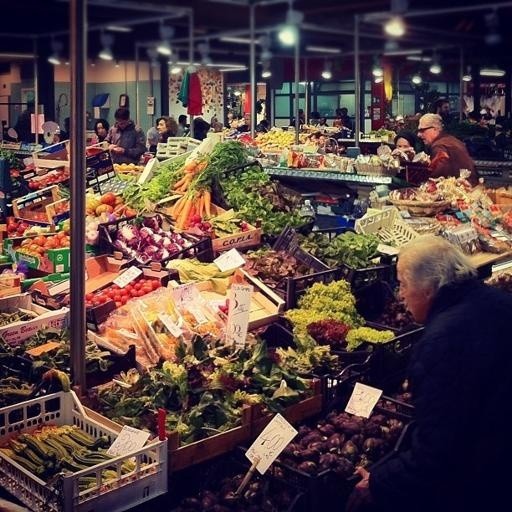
[172,161,211,230]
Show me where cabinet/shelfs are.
[0,126,512,512]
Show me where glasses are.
[417,127,433,134]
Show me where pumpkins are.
[86,193,137,218]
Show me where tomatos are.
[85,277,159,308]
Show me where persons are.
[341,232,512,512]
[102,107,147,166]
[415,113,479,188]
[10,99,71,154]
[466,105,504,142]
[155,116,178,144]
[429,99,450,119]
[175,107,355,143]
[394,132,415,149]
[94,118,110,146]
[146,126,160,156]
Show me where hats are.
[393,129,418,148]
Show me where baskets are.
[388,189,450,217]
[355,161,397,176]
[97,214,213,274]
[234,206,420,512]
[0,389,169,512]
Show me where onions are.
[20,234,71,254]
[181,391,414,512]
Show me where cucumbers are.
[9,425,135,489]
